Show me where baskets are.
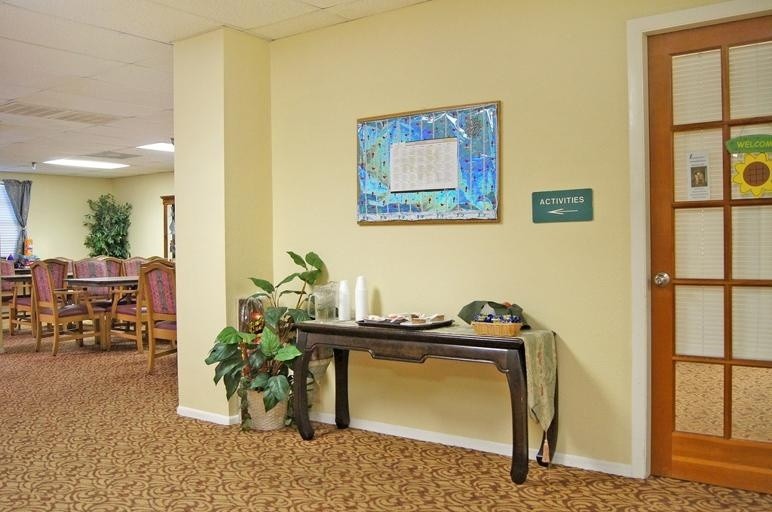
[472,322,522,337]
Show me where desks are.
[294,316,558,484]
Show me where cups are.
[335,279,352,321]
[354,275,369,323]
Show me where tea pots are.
[307,281,339,323]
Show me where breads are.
[410,314,445,324]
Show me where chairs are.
[0,255,177,373]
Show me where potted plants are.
[206,251,338,432]
